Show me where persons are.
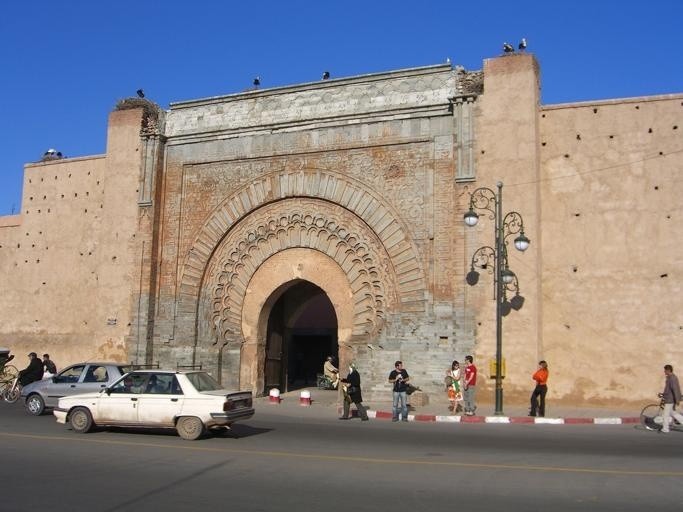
[339,364,369,420]
[19,352,44,386]
[324,357,339,389]
[463,355,477,416]
[389,361,409,422]
[660,365,683,432]
[528,361,549,417]
[447,361,463,415]
[43,354,57,379]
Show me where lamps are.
[499,38,529,55]
[250,72,265,91]
[319,71,330,81]
[41,147,63,160]
[135,88,145,100]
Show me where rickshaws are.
[0,349,20,400]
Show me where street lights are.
[466,180,531,417]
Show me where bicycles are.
[641,392,683,434]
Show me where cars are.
[52,367,258,439]
[19,359,141,422]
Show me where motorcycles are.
[316,370,341,389]
[2,371,21,405]
